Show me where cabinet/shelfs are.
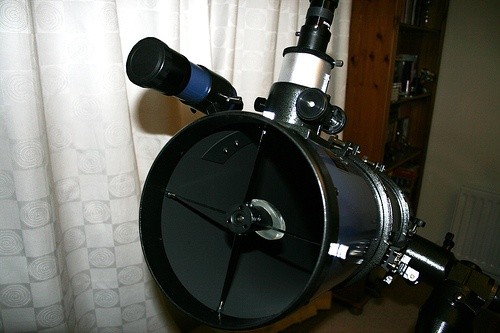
[342,0,451,227]
